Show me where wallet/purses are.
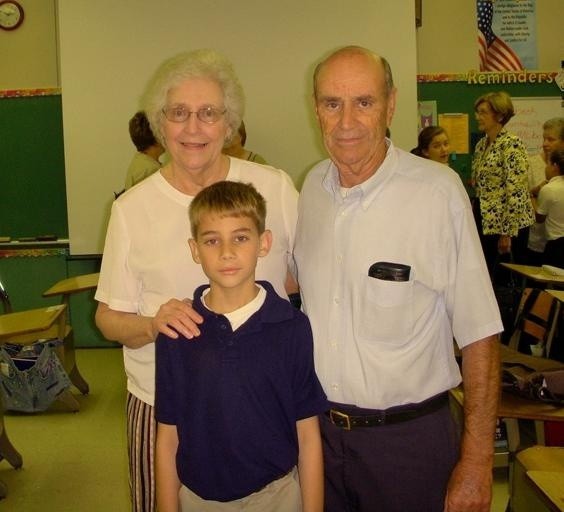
[369,262,411,281]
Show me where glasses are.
[163,105,228,123]
[474,110,490,116]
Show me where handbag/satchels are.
[0,338,71,412]
[495,251,521,319]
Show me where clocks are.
[1,1,25,32]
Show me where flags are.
[477,0,524,74]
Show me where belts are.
[325,393,450,429]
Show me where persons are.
[95,48,301,511]
[221,118,270,170]
[121,111,165,191]
[465,89,537,342]
[407,124,452,168]
[291,46,505,511]
[527,115,563,266]
[529,148,564,290]
[150,181,330,511]
